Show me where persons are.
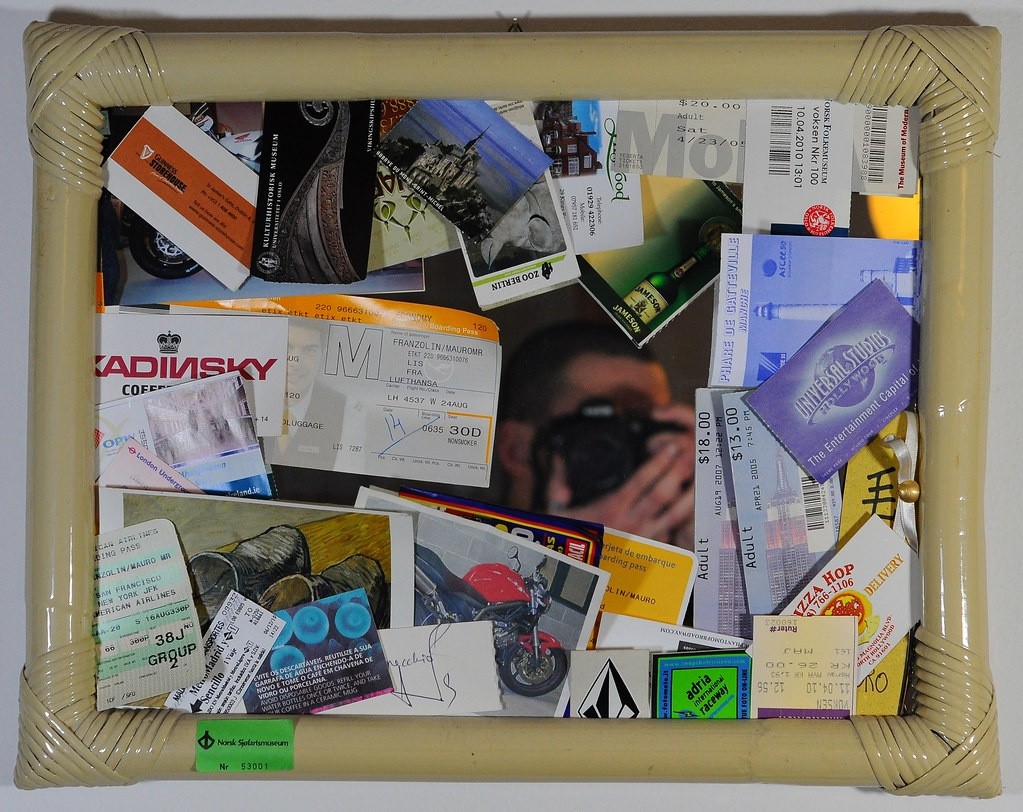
[486,304,695,552]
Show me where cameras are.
[532,396,688,508]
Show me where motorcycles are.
[414,541,569,699]
